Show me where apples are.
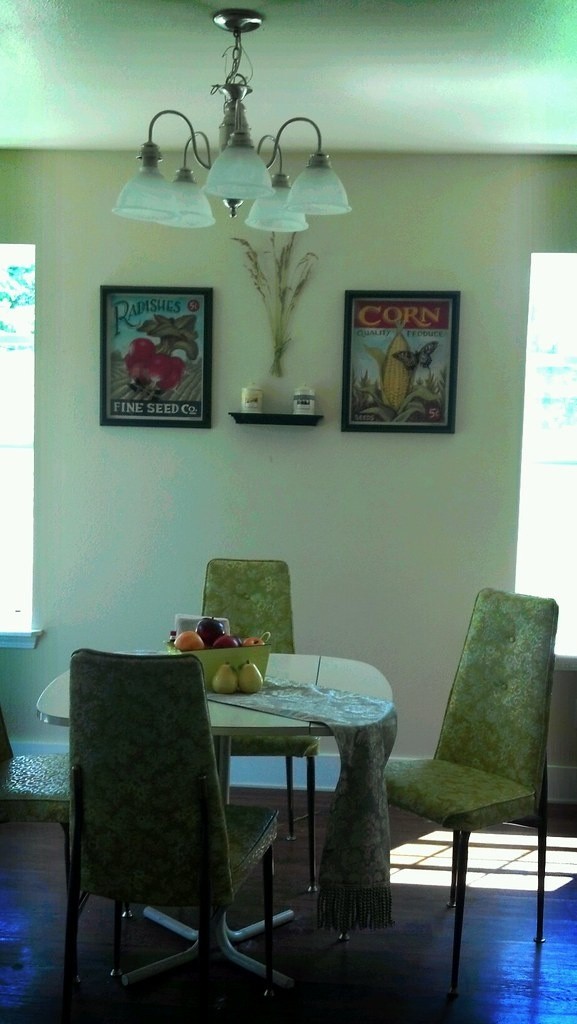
[173,617,265,651]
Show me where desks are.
[36,653,394,991]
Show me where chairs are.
[384,588,559,999]
[62,648,280,1023]
[201,558,321,894]
[0,705,133,984]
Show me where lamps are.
[111,8,352,232]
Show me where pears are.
[212,659,264,694]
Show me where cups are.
[242,381,263,413]
[293,383,316,414]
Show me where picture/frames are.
[341,289,460,434]
[99,285,213,428]
